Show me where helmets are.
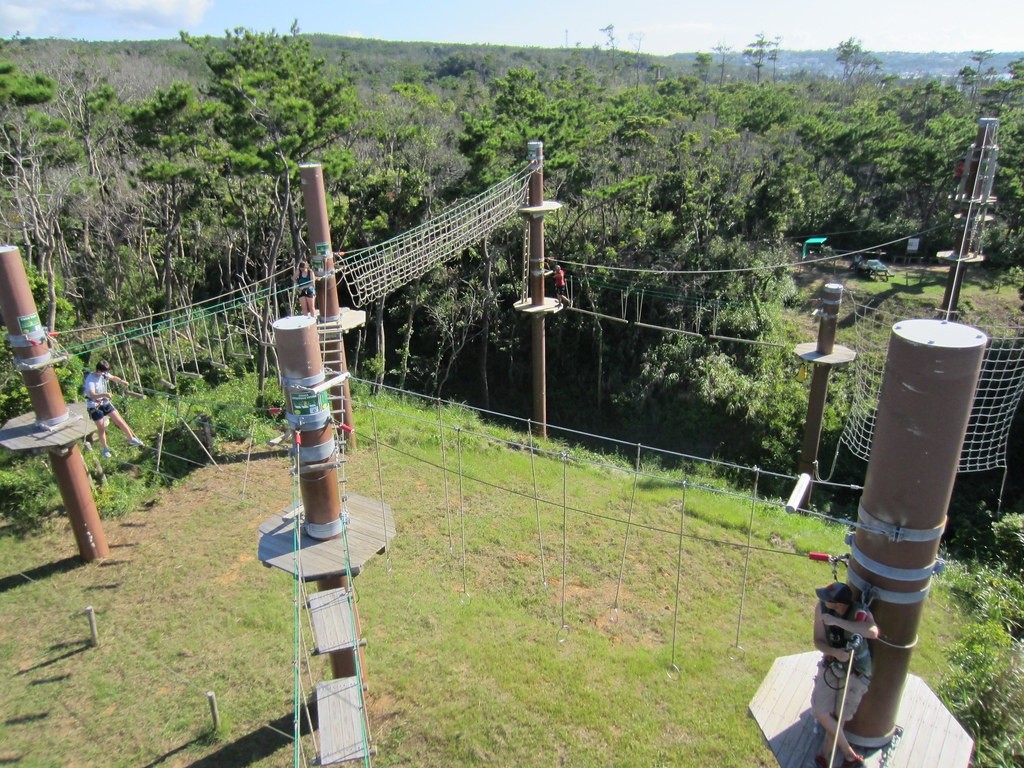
[554,265,561,270]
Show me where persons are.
[291,262,316,318]
[847,252,871,279]
[552,265,570,307]
[950,160,964,199]
[265,402,286,421]
[85,360,142,458]
[811,582,878,768]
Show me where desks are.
[868,259,889,282]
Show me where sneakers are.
[103,449,111,458]
[840,754,867,768]
[130,438,143,446]
[814,755,828,768]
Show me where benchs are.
[860,262,894,280]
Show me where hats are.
[815,583,852,602]
